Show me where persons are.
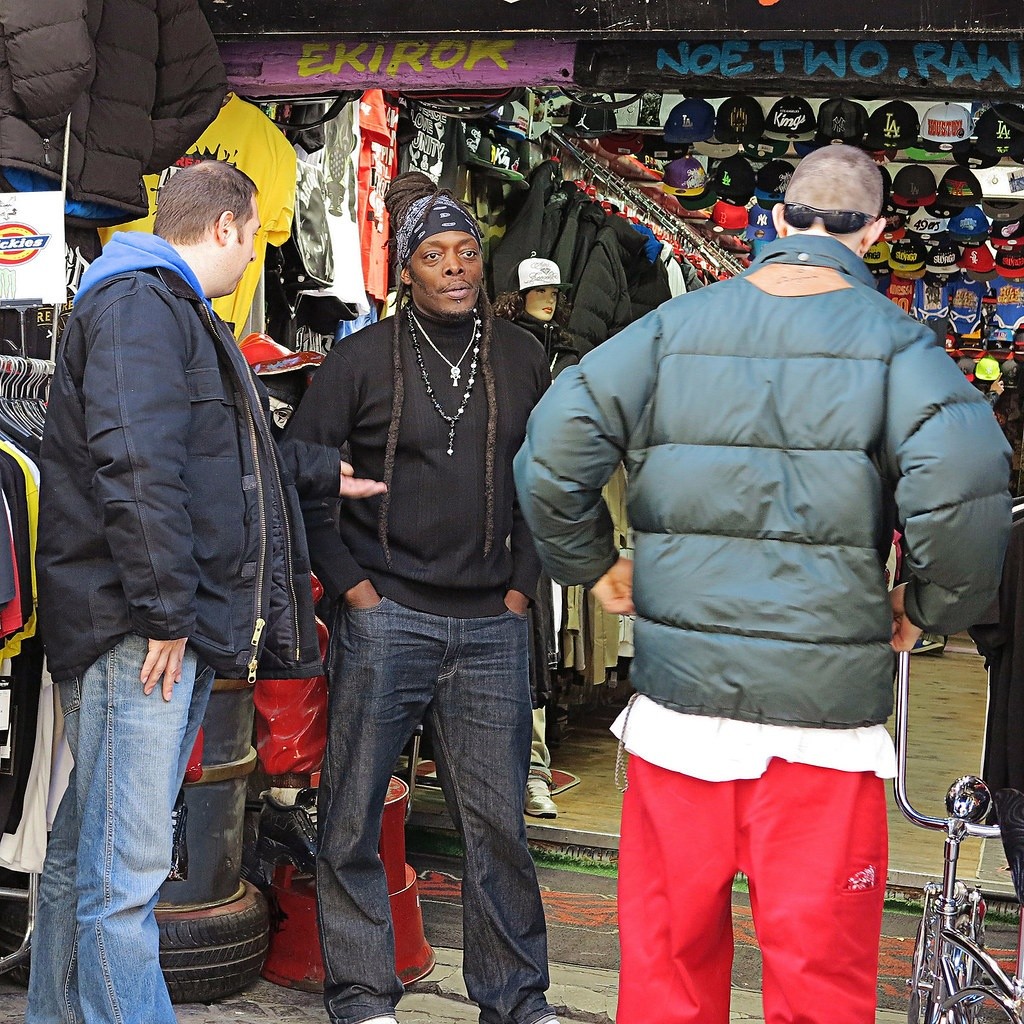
[34,161,319,1024]
[910,372,1004,653]
[280,169,553,1023]
[492,257,579,813]
[512,144,1013,1023]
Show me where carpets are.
[404,826,1023,1023]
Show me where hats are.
[464,87,1024,389]
[505,251,574,296]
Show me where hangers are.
[553,145,745,285]
[0,355,56,453]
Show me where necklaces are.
[405,301,481,457]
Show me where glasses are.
[784,202,876,236]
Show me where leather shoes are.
[254,794,319,877]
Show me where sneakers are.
[911,639,945,655]
[524,778,558,819]
